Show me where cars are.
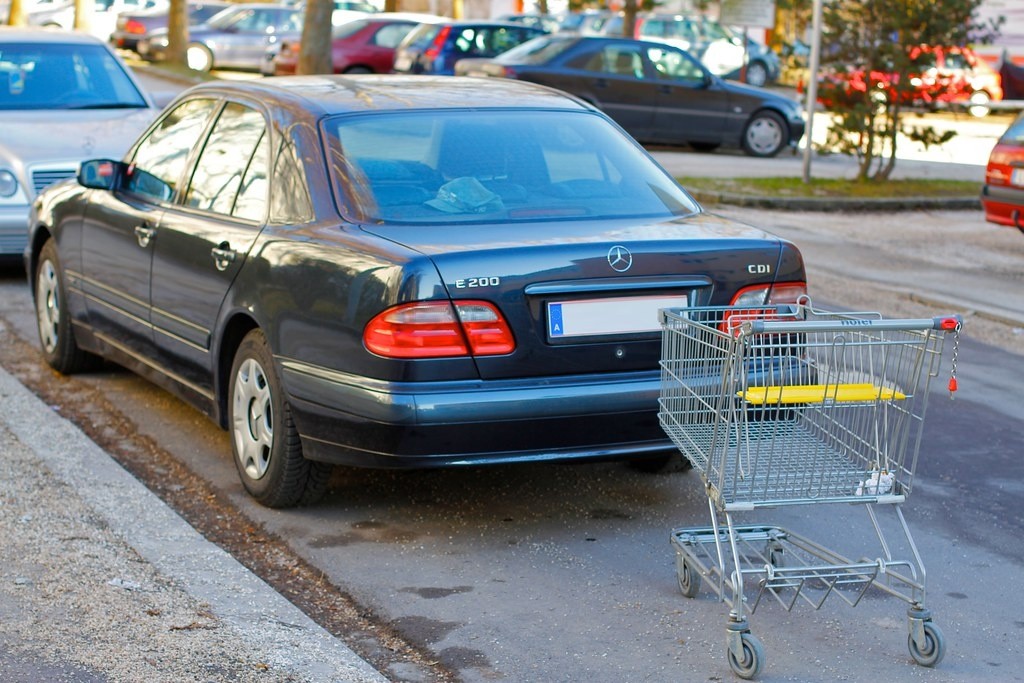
[460,35,805,157]
[26,74,815,511]
[1,27,160,257]
[0,0,777,87]
[797,46,1005,118]
[980,109,1024,235]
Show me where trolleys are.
[657,292,963,678]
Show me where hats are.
[423,177,506,214]
